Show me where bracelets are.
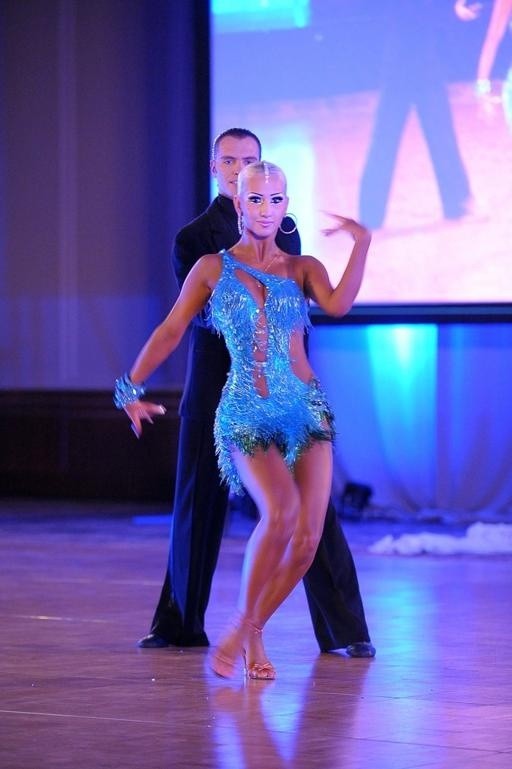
[474,79,492,96]
[114,372,147,410]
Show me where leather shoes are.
[347,641,376,657]
[137,634,177,648]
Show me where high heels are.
[209,616,276,680]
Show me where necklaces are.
[256,249,280,288]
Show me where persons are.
[112,162,371,679]
[359,0,474,233]
[475,0,512,128]
[137,128,376,658]
